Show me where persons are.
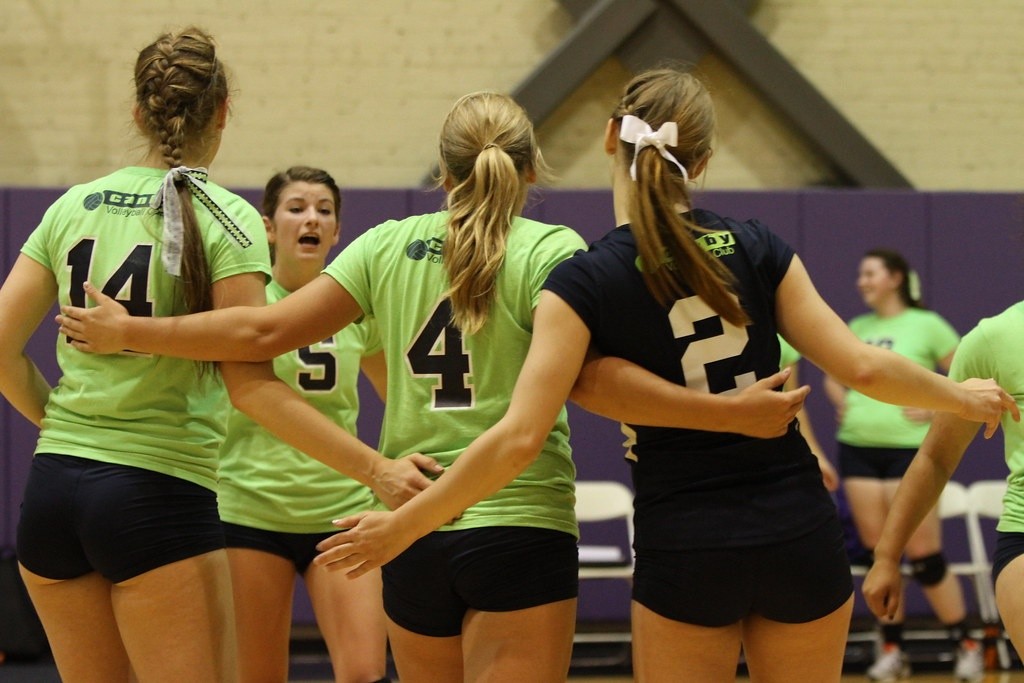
[861,300,1024,683]
[0,22,462,683]
[216,165,387,683]
[822,248,984,683]
[777,332,840,492]
[54,91,811,683]
[313,69,1020,683]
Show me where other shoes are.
[866,642,910,683]
[955,637,983,683]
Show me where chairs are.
[569,477,1015,669]
